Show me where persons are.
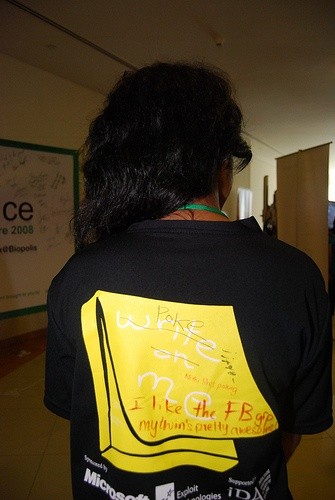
[328,219,335,316]
[263,189,278,238]
[43,58,334,500]
